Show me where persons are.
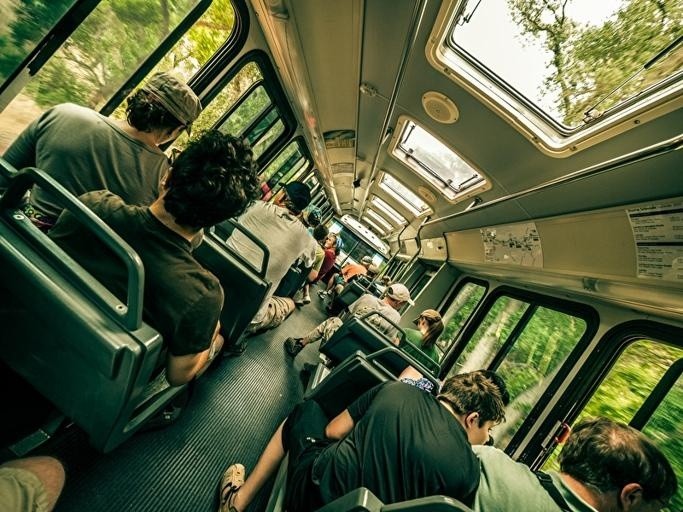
[397,365,509,407]
[284,283,415,358]
[395,309,443,371]
[0,456,66,512]
[0,70,204,252]
[293,208,391,306]
[46,130,263,386]
[219,371,506,512]
[225,181,318,356]
[471,415,678,512]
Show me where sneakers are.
[317,289,328,300]
[285,336,305,358]
[294,296,311,307]
[218,462,246,512]
[235,337,249,356]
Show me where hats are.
[278,181,312,210]
[382,276,391,281]
[368,263,381,273]
[303,203,322,227]
[360,255,372,265]
[335,235,345,248]
[412,309,442,327]
[142,71,203,138]
[387,284,415,307]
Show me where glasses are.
[168,148,182,167]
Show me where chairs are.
[328,277,386,309]
[302,346,443,414]
[273,257,308,299]
[194,218,272,358]
[0,165,192,461]
[317,487,475,511]
[0,158,18,194]
[318,311,441,378]
